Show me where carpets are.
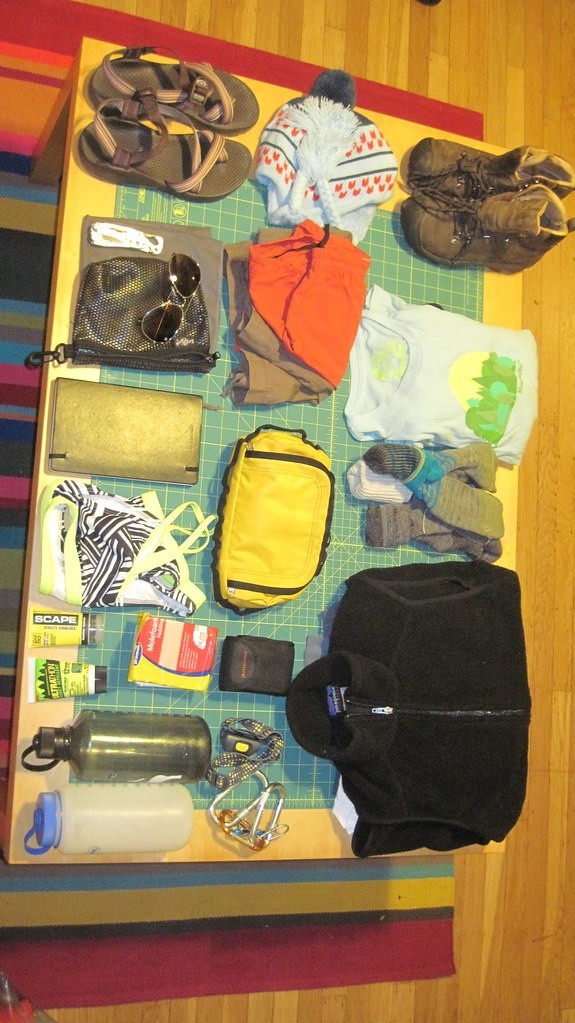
[0,0,488,1010]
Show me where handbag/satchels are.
[214,425,335,616]
[27,258,221,375]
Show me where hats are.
[255,70,398,246]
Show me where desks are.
[5,34,524,871]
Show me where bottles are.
[33,709,212,784]
[23,783,193,856]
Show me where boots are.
[400,185,575,275]
[407,137,575,203]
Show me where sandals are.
[78,98,252,202]
[89,46,259,134]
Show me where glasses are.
[137,252,201,345]
